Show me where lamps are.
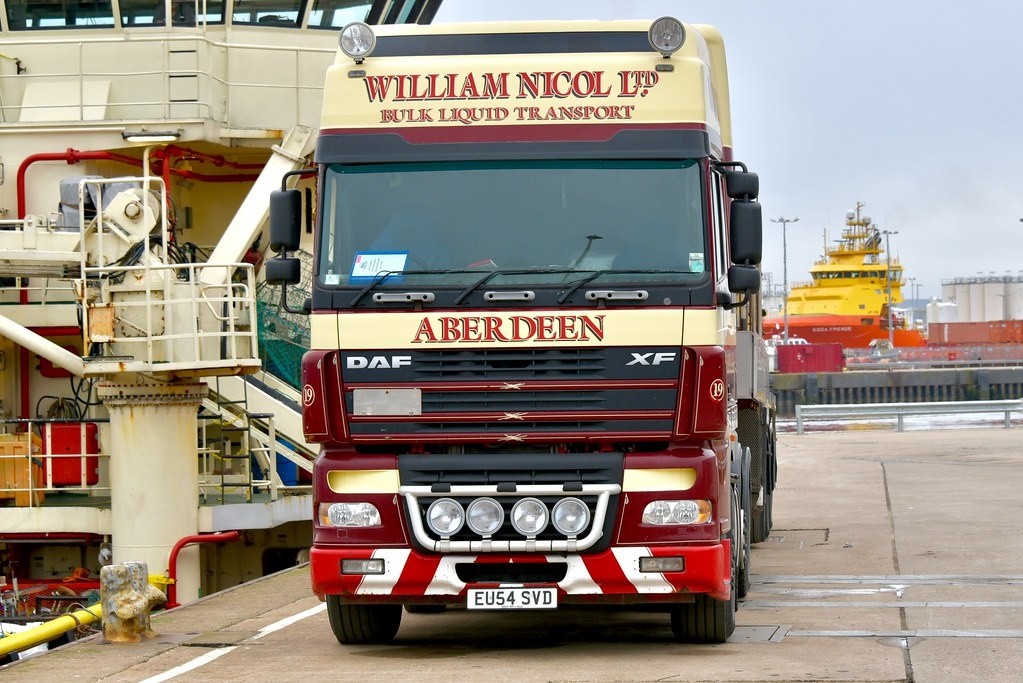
[97,536,113,565]
[122,132,183,143]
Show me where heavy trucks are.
[267,16,778,644]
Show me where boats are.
[760,202,925,348]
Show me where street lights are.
[883,230,899,347]
[769,218,799,344]
[908,277,916,309]
